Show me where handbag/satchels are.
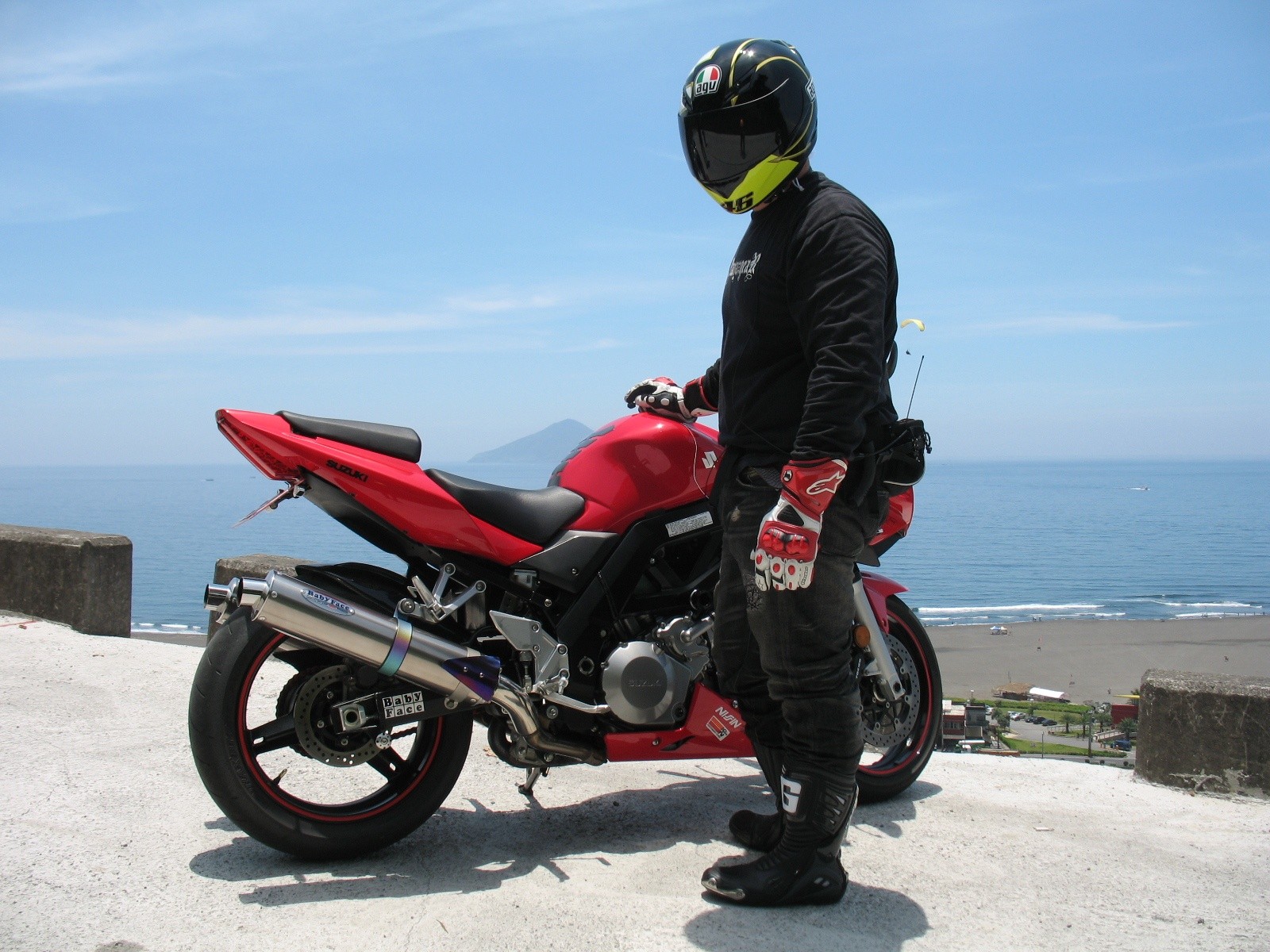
[877,417,927,496]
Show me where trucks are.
[955,740,986,753]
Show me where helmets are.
[677,38,818,214]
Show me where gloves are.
[624,375,718,423]
[750,456,848,592]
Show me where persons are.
[624,37,897,908]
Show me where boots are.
[701,766,858,906]
[729,728,787,853]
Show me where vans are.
[1115,739,1133,751]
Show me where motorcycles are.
[188,339,943,864]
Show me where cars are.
[985,705,994,715]
[1007,711,1058,726]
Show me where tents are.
[989,625,1008,635]
